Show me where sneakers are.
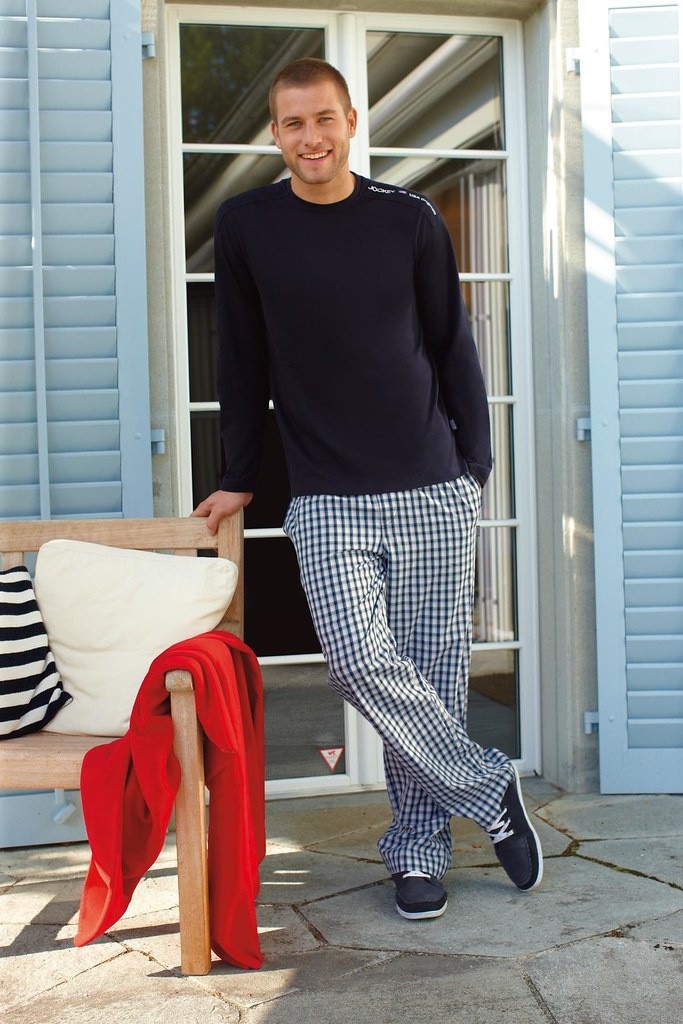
[391,871,448,919]
[483,760,544,892]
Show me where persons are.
[191,57,544,920]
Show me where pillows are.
[30,540,239,740]
[0,565,73,740]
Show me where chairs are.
[0,506,244,976]
